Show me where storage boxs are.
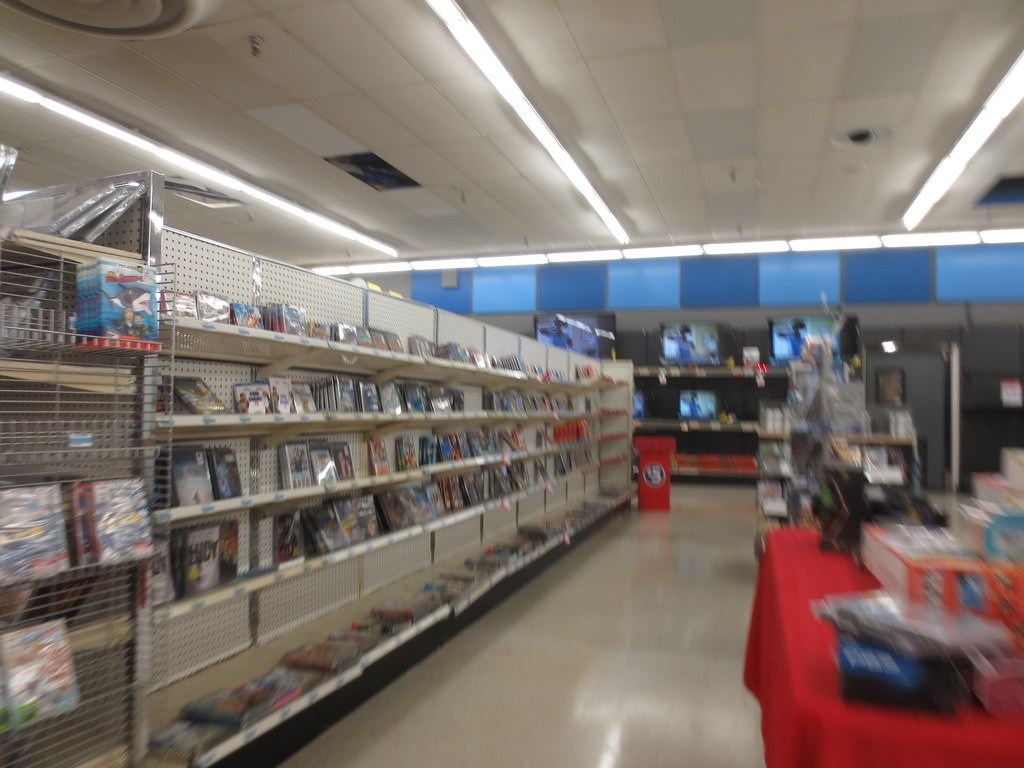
[859,449,1024,658]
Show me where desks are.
[744,527,1023,768]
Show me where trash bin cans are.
[632,434,676,513]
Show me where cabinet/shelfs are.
[634,367,790,560]
[0,170,634,767]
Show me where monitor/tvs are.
[769,314,858,366]
[677,388,721,421]
[533,314,617,360]
[632,390,645,419]
[661,320,723,367]
